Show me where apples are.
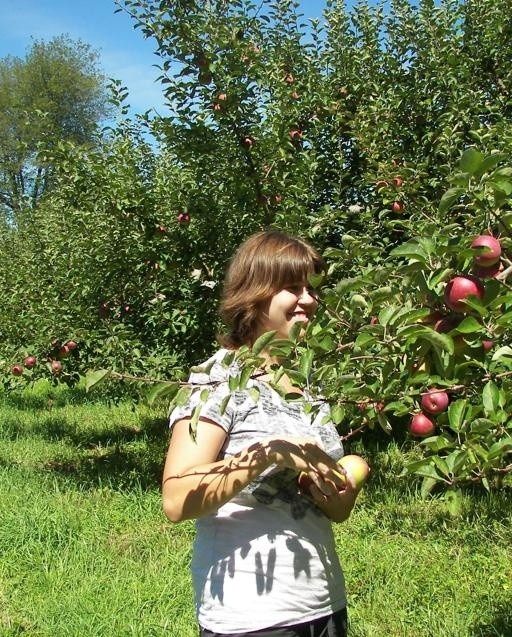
[12,341,78,376]
[297,454,369,494]
[198,46,303,205]
[178,212,191,223]
[371,235,506,439]
[376,159,404,215]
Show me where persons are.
[162,231,375,634]
